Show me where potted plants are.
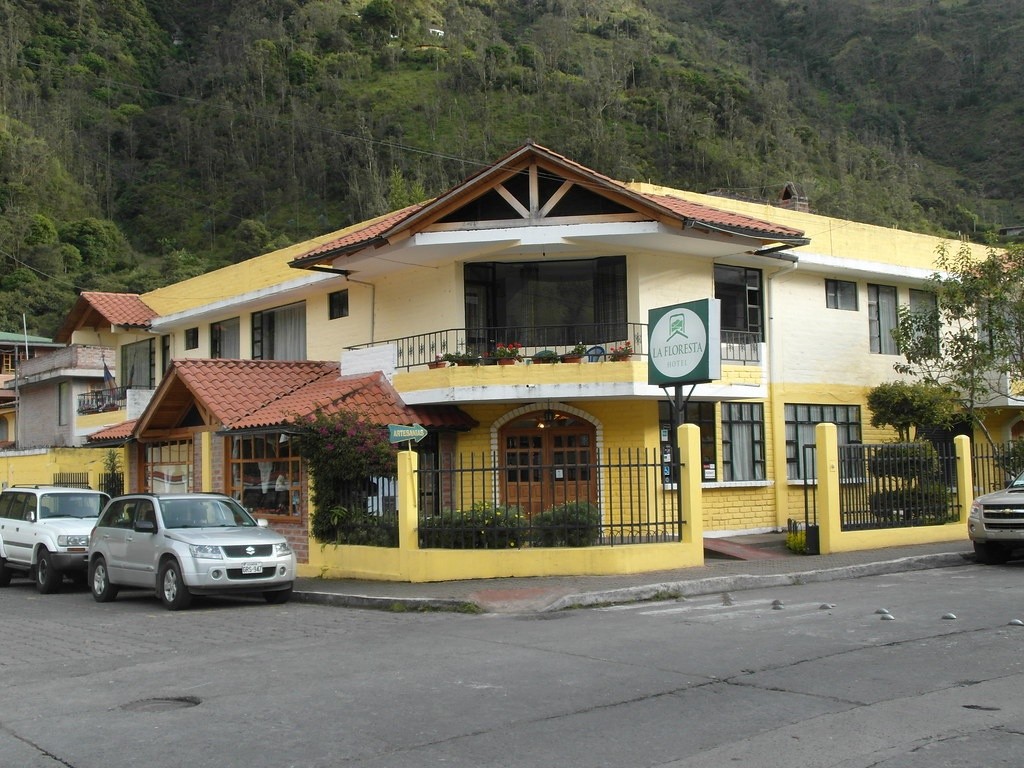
[532,350,561,364]
[443,352,482,366]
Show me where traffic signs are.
[388,422,429,444]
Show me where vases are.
[564,355,582,363]
[428,362,446,369]
[620,357,630,361]
[83,410,100,414]
[102,407,118,412]
[484,357,497,365]
[497,357,516,365]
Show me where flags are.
[104,366,118,395]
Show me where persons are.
[275,471,294,511]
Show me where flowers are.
[104,401,119,408]
[493,342,524,362]
[76,404,90,414]
[608,341,633,363]
[434,354,444,362]
[566,341,587,355]
[483,352,489,358]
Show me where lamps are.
[544,399,555,423]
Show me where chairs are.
[164,503,187,522]
[70,506,95,517]
[585,346,604,362]
[190,504,209,522]
[145,510,158,528]
[41,506,50,518]
[125,507,135,525]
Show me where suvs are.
[966,469,1024,566]
[0,484,125,594]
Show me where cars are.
[86,491,298,611]
